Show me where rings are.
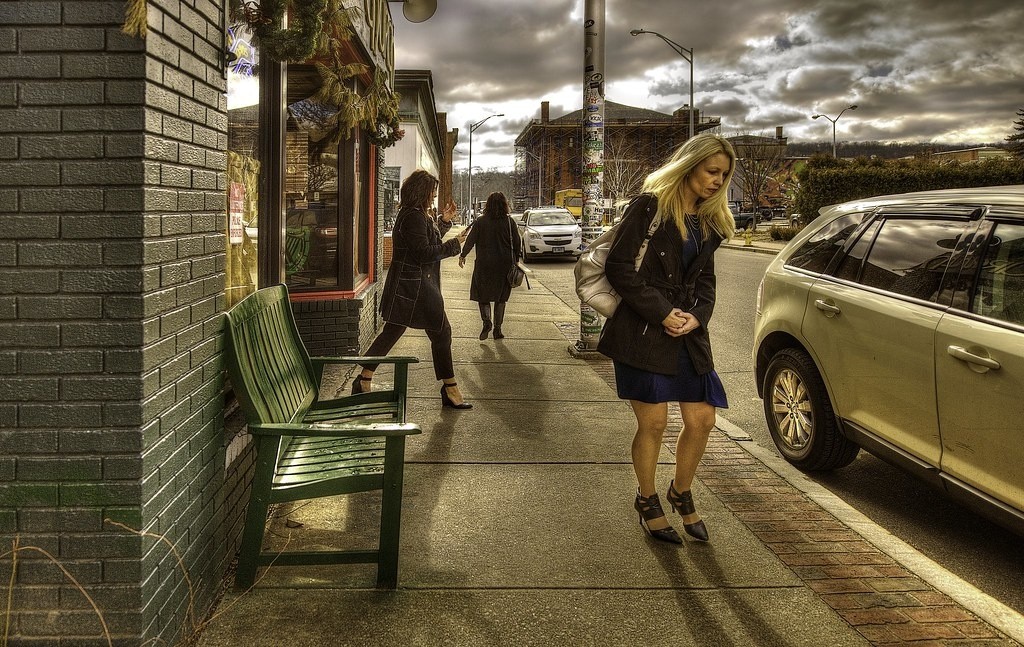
[682,327,684,333]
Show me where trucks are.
[555,189,607,229]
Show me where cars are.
[516,207,583,263]
[750,185,1023,534]
[509,213,523,224]
[473,201,487,222]
[456,206,464,214]
[471,202,475,217]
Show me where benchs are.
[224,282,422,594]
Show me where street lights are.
[468,114,506,225]
[630,28,695,140]
[812,104,858,159]
[518,149,543,208]
[509,175,533,209]
[459,165,481,225]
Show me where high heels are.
[667,480,709,541]
[441,383,473,409]
[634,486,682,544]
[351,374,372,395]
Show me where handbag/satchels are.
[507,263,530,290]
[574,193,661,318]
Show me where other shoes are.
[493,328,504,340]
[480,319,492,340]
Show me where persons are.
[351,170,473,409]
[596,133,735,543]
[458,191,522,341]
